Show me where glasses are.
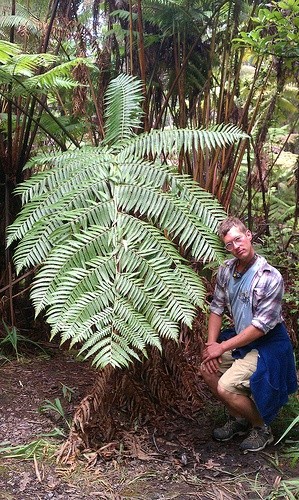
[223,233,246,251]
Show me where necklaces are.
[233,254,257,278]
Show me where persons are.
[199,218,298,451]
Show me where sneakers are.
[212,419,248,441]
[239,425,274,451]
